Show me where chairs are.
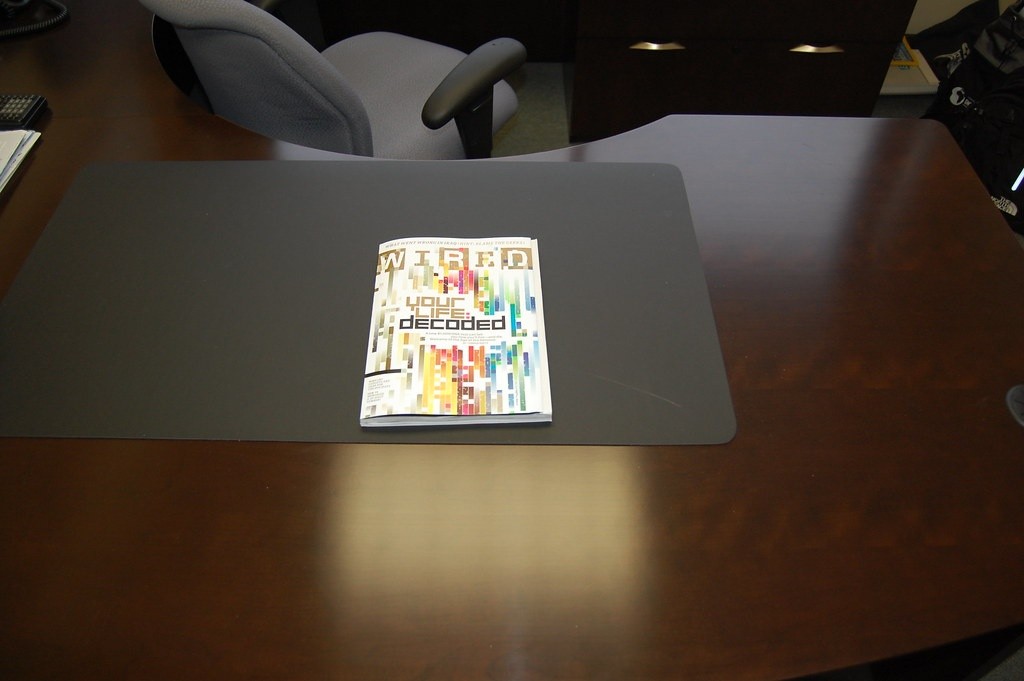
[138,0,528,159]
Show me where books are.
[360,236,553,427]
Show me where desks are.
[0,0,1024,681]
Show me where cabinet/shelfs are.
[562,0,917,144]
